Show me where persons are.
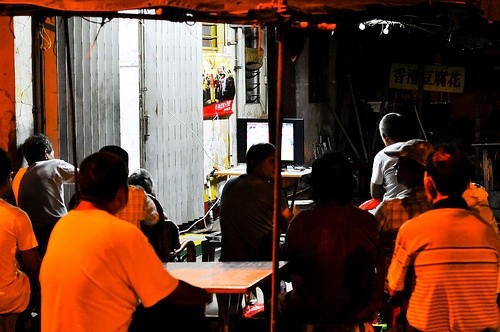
[215,112,500,332]
[0,133,213,332]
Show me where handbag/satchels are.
[146,194,180,251]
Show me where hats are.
[246,144,275,175]
[385,140,435,168]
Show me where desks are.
[213,165,312,213]
[161,260,287,332]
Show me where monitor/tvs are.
[236,118,305,166]
